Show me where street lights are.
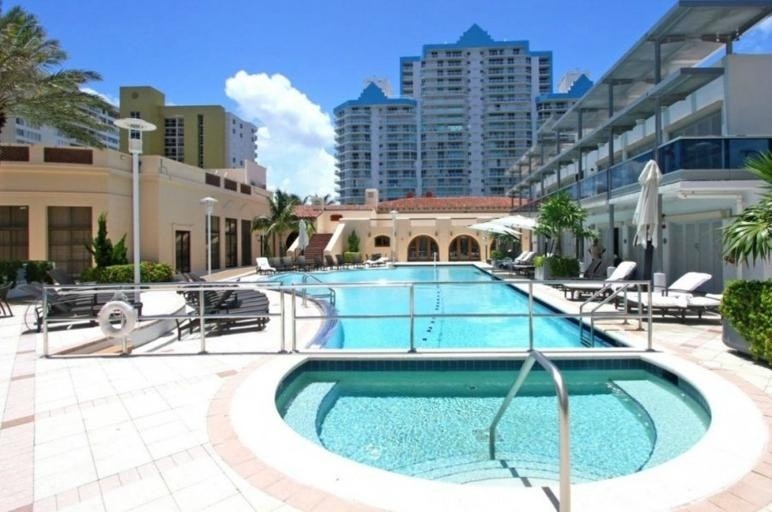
[111,116,159,303]
[198,196,219,276]
[388,210,399,259]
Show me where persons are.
[0,261,18,290]
[585,235,607,263]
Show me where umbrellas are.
[466,213,561,259]
[297,219,310,256]
[631,158,665,292]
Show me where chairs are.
[34,271,270,342]
[497,250,536,273]
[543,260,725,322]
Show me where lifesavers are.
[98,301,136,339]
